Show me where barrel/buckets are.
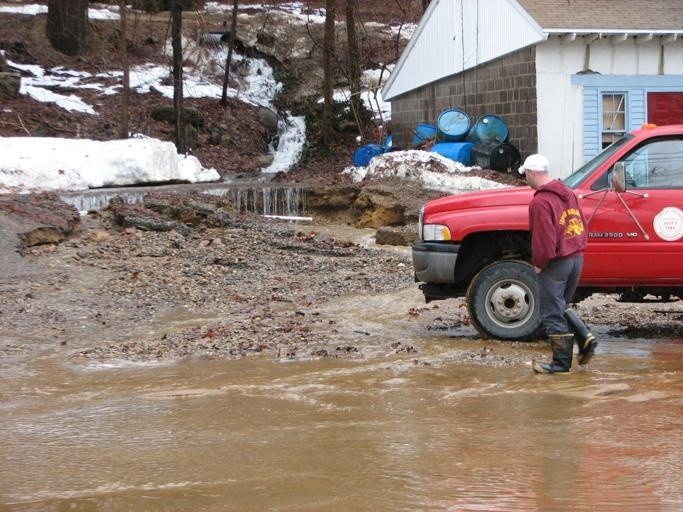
[352,108,522,174]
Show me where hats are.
[518,154,549,175]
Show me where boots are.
[563,308,598,365]
[533,333,575,375]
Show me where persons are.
[519,153,598,377]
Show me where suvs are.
[410,121,682,343]
